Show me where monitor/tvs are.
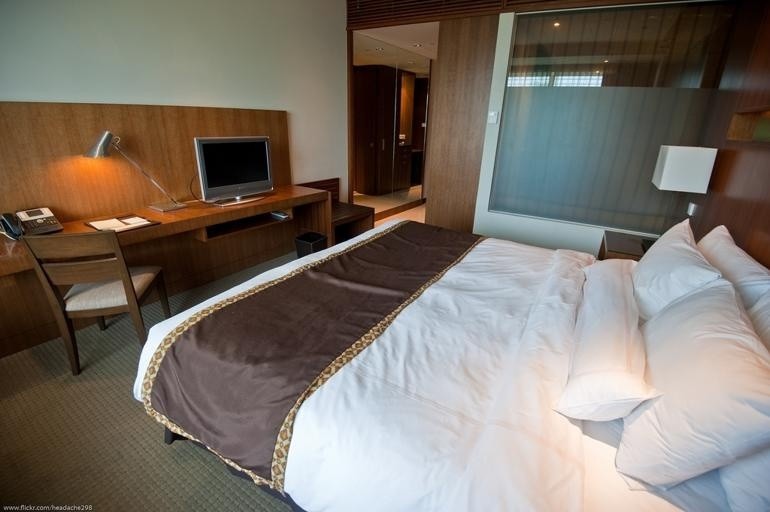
[193,135,274,208]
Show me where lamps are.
[83,130,188,212]
[651,145,718,194]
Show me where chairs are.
[18,229,171,375]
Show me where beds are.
[133,218,770,512]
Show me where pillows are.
[718,285,770,512]
[553,257,661,423]
[698,225,770,309]
[613,278,770,493]
[633,219,723,323]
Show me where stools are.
[332,199,374,242]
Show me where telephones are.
[1,206,65,238]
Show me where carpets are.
[0,250,301,511]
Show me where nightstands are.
[595,230,660,262]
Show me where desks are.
[1,184,333,358]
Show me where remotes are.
[273,211,288,220]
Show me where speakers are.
[293,230,327,258]
